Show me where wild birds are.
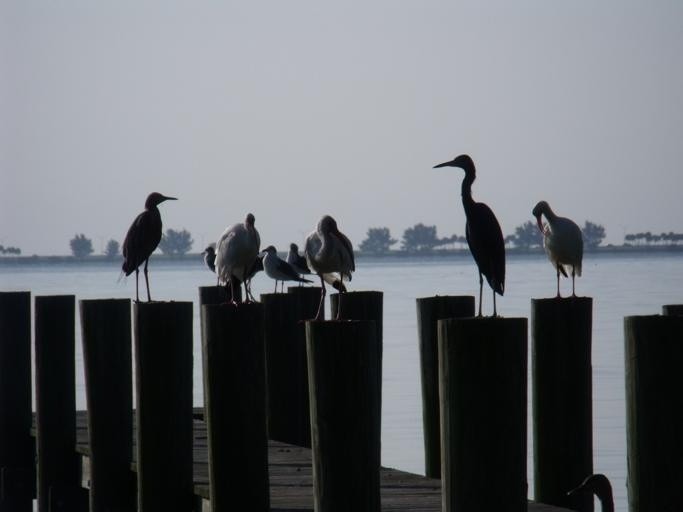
[286,242,314,288]
[566,474,614,512]
[532,200,583,297]
[305,214,356,321]
[262,244,314,294]
[205,246,264,303]
[213,213,261,303]
[117,191,179,302]
[433,153,506,319]
